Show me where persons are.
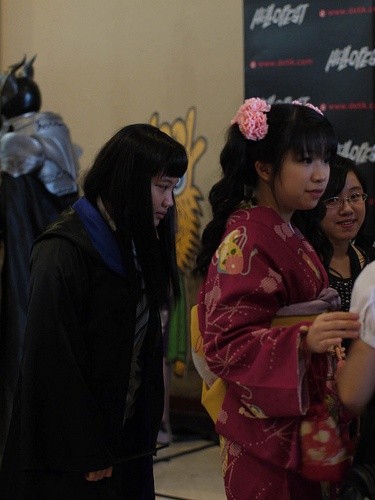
[191,97,375,500]
[0,54,80,440]
[0,123,189,500]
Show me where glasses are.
[322,192,368,209]
